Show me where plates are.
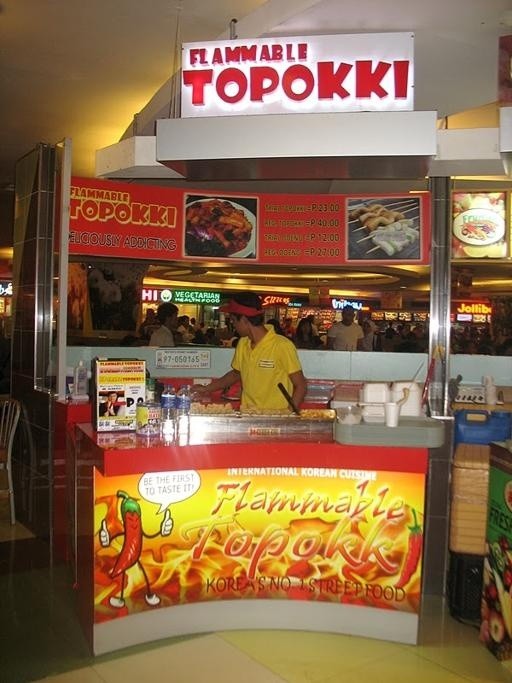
[453,207,506,246]
[186,197,257,258]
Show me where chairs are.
[0,396,20,528]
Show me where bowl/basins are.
[334,404,363,424]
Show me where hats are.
[218,298,264,316]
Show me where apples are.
[480,535,512,648]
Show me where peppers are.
[393,508,423,588]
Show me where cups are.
[485,383,497,405]
[384,403,400,428]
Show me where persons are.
[99,391,120,416]
[140,301,512,358]
[189,291,308,411]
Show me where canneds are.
[136,402,162,437]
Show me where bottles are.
[158,383,191,436]
[72,360,90,397]
